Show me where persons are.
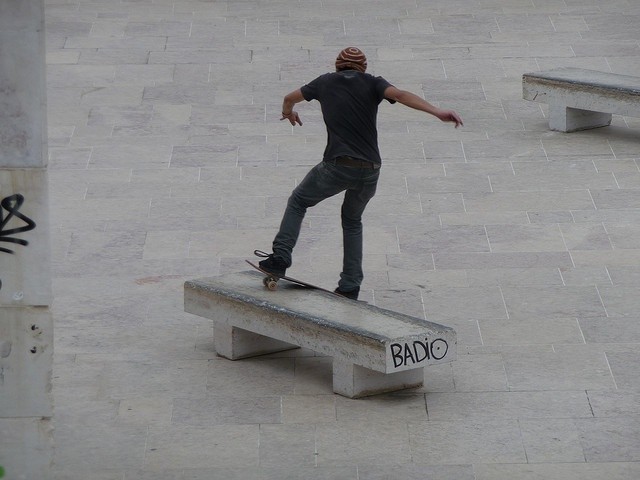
[256,47,463,301]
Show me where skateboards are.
[244,259,369,303]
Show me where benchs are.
[182,269,458,400]
[522,66,639,134]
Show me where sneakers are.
[254,249,288,276]
[334,286,360,301]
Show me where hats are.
[335,47,368,74]
